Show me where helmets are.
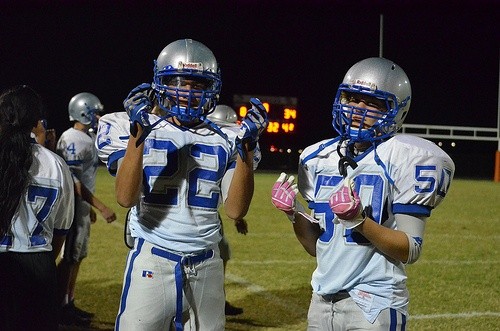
[334,56,411,140]
[153,39,219,121]
[68,93,104,125]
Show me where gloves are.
[235,97,269,159]
[270,172,318,225]
[122,82,152,147]
[328,177,367,229]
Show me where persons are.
[0,39,268,331]
[271,58,455,331]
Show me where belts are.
[151,247,212,330]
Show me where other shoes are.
[60,302,91,327]
[68,298,94,319]
[225,301,243,315]
